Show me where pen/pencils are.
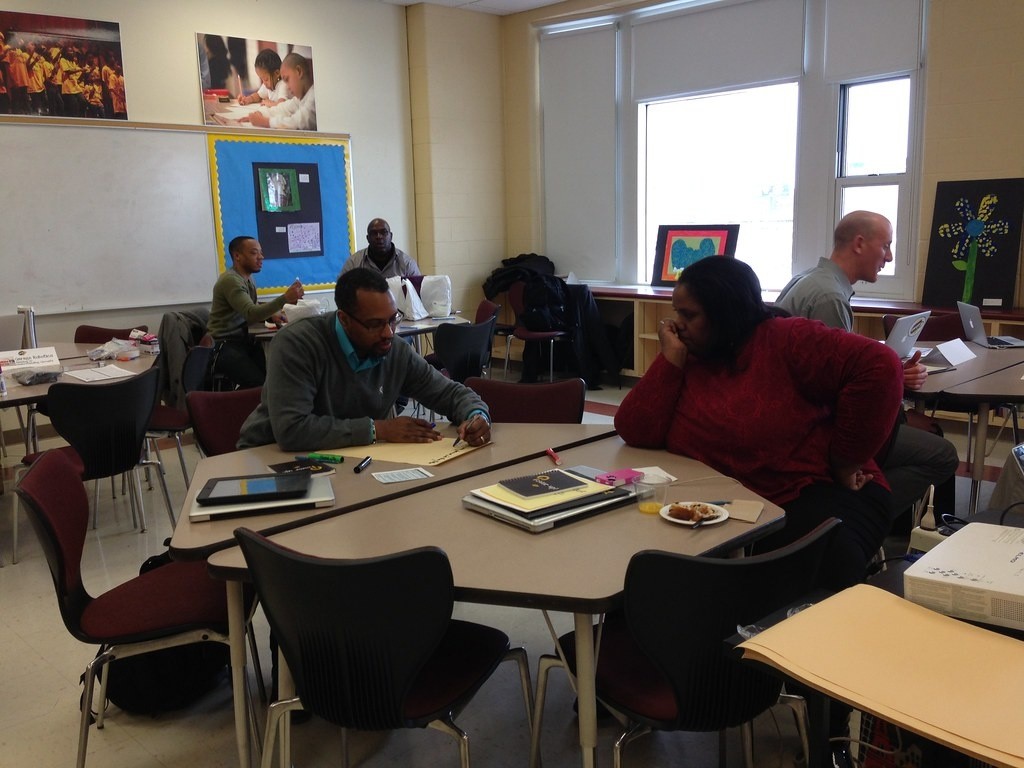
[452,412,481,447]
[399,325,418,329]
[295,455,337,463]
[706,500,732,505]
[432,317,455,320]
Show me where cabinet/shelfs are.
[632,298,674,380]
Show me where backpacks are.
[520,302,571,367]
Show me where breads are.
[669,502,719,522]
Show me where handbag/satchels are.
[87,551,230,716]
[401,278,429,321]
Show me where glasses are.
[340,308,404,333]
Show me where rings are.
[481,436,484,442]
[659,320,665,325]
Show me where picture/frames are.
[207,133,356,295]
[652,224,743,286]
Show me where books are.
[267,456,336,478]
[0,347,63,381]
[498,469,586,499]
[116,350,140,361]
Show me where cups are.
[632,474,673,515]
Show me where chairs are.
[157,309,229,390]
[119,332,217,491]
[430,315,497,422]
[10,448,263,768]
[184,389,263,460]
[529,515,844,768]
[74,325,149,344]
[503,282,569,383]
[231,525,541,768]
[474,299,503,383]
[883,311,1021,473]
[464,377,586,423]
[12,365,176,565]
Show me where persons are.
[197,31,317,132]
[613,255,904,768]
[0,28,128,120]
[236,266,491,447]
[775,210,960,569]
[207,236,304,387]
[338,218,420,281]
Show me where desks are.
[0,292,1024,768]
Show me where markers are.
[307,452,346,464]
[281,316,285,321]
[546,447,562,466]
[354,457,373,473]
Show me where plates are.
[659,501,730,526]
